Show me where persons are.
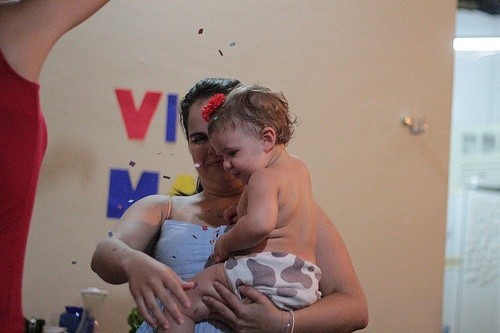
[146,82,321,333]
[0,1,115,333]
[90,76,370,333]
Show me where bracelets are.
[280,309,296,333]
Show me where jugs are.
[76,286,108,333]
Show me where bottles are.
[59,306,93,333]
